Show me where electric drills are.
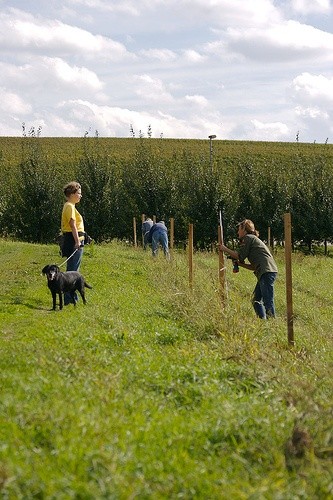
[227,255,239,273]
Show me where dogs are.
[40,263,94,312]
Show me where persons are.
[219,219,279,318]
[142,218,156,251]
[61,182,86,306]
[149,221,170,261]
